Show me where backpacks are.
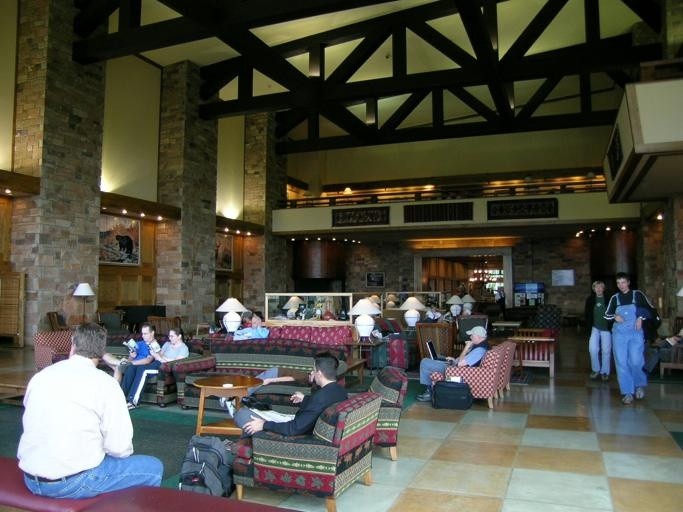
[176,433,235,500]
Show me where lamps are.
[458,291,475,316]
[344,293,398,338]
[71,282,94,324]
[282,296,304,318]
[397,297,426,328]
[215,296,250,334]
[444,294,464,318]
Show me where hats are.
[465,326,488,338]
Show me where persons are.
[114,321,161,387]
[397,277,506,324]
[121,325,189,410]
[16,322,164,501]
[218,352,348,436]
[581,272,616,382]
[232,311,270,340]
[643,287,683,381]
[415,326,488,403]
[603,272,656,405]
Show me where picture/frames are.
[96,211,145,270]
[213,230,235,273]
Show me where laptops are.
[425,341,450,363]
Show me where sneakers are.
[127,401,140,410]
[589,371,609,381]
[416,390,432,402]
[635,387,645,400]
[621,393,634,405]
[119,357,133,368]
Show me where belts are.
[24,469,86,483]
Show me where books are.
[149,339,161,352]
[122,338,138,352]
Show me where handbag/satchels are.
[430,380,475,410]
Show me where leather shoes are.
[239,395,272,411]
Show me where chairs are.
[656,342,682,381]
